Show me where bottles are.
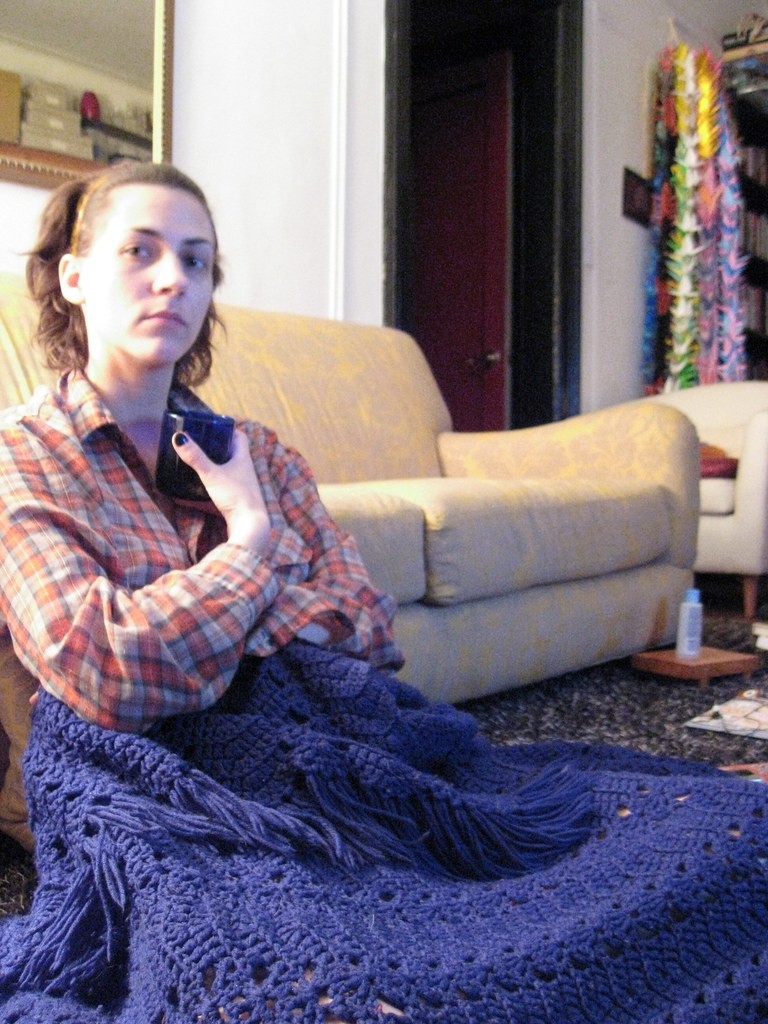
[677,590,703,661]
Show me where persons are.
[1,160,405,730]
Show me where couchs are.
[645,377,767,617]
[1,278,700,854]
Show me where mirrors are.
[0,0,174,193]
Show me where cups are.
[155,409,233,501]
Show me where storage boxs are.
[0,71,95,159]
